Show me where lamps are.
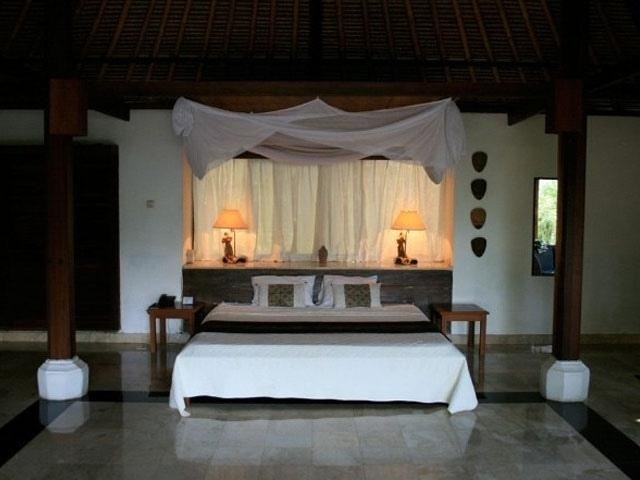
[212,209,248,263]
[390,210,426,265]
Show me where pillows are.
[250,274,382,309]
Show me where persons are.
[396,232,407,258]
[221,232,234,257]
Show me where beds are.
[176,300,468,409]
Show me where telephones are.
[158,294,176,308]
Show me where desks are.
[146,301,206,353]
[429,303,490,355]
